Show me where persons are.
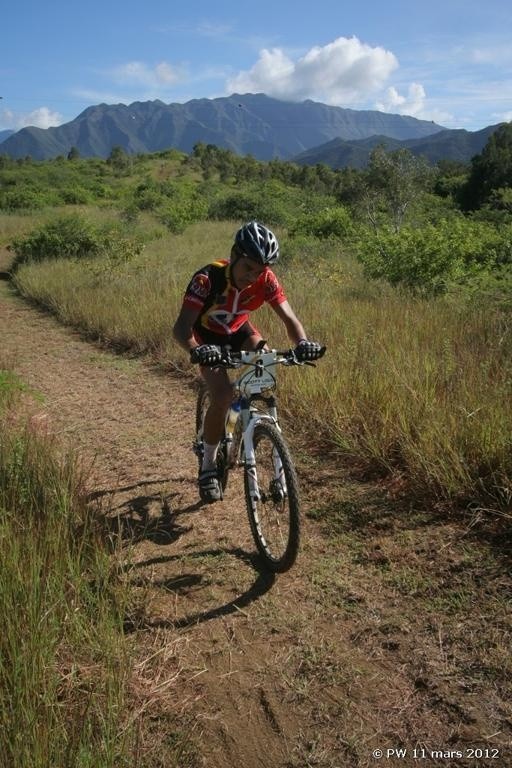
[170,218,322,504]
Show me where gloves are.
[293,339,321,363]
[190,343,222,367]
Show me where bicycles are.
[186,343,324,574]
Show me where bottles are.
[225,398,242,434]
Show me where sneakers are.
[196,463,221,501]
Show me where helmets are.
[236,221,280,267]
